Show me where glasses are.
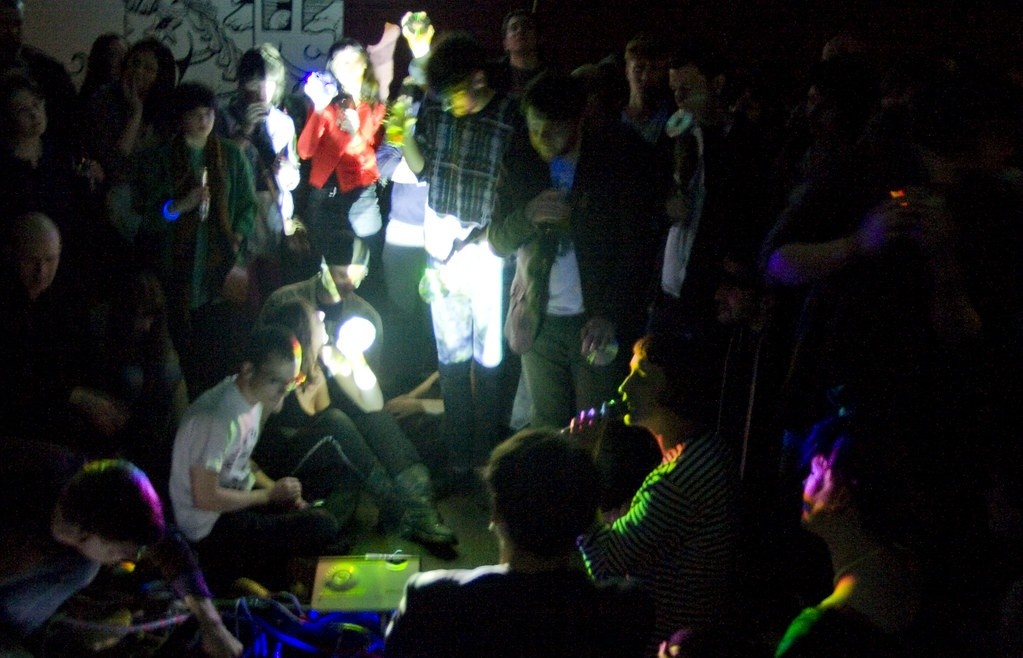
[267,373,307,391]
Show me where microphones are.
[560,400,626,436]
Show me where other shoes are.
[399,499,458,543]
[448,458,474,482]
[376,488,405,520]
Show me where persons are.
[296,35,387,253]
[0,8,1022,658]
